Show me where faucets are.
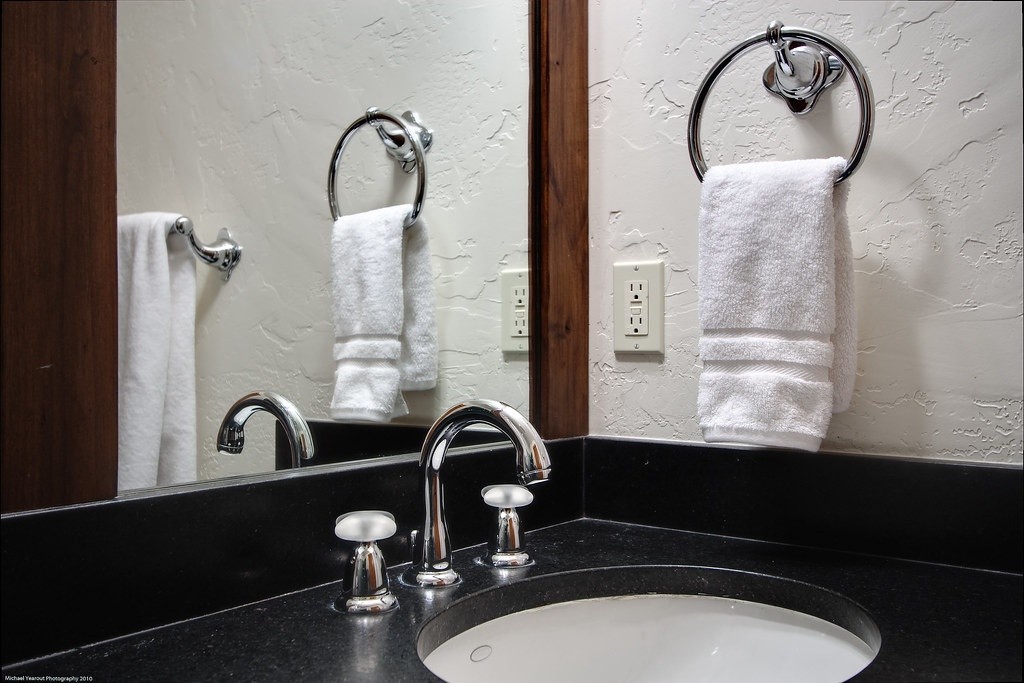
[216,388,317,469]
[399,397,556,589]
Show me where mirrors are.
[118,0,530,491]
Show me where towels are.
[117,210,200,493]
[328,201,443,426]
[693,154,863,451]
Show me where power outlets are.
[612,261,666,354]
[500,268,529,353]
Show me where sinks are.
[414,561,884,683]
